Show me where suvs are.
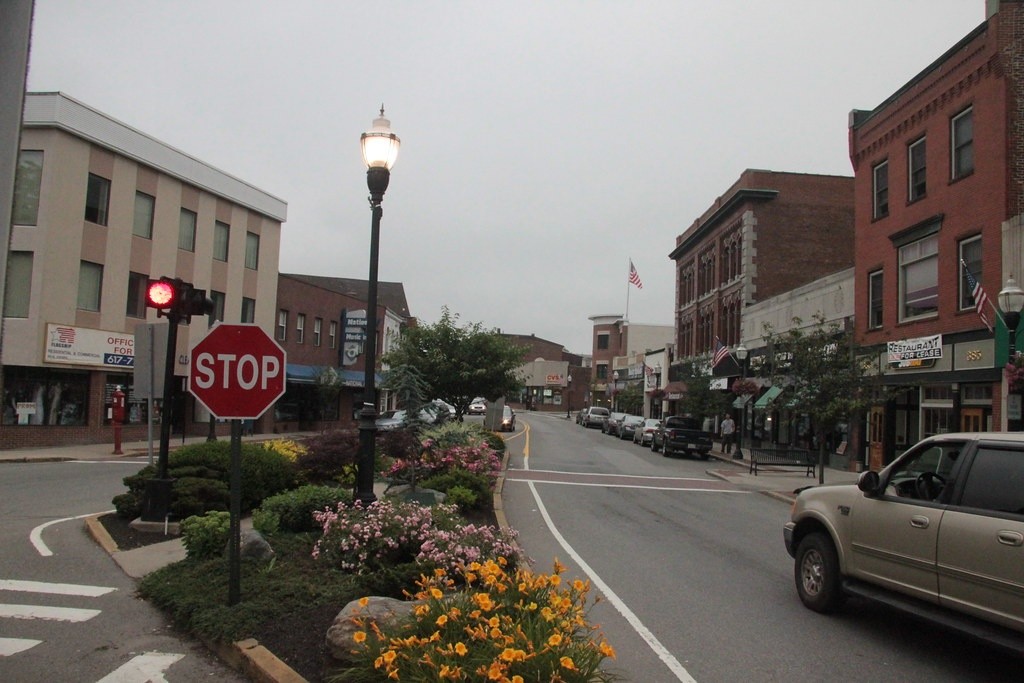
[780,431,1024,664]
[580,406,609,428]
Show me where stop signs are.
[187,322,286,421]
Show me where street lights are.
[353,102,401,503]
[613,370,619,412]
[566,374,572,418]
[997,272,1023,427]
[653,360,661,416]
[733,338,749,459]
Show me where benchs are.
[750,447,817,479]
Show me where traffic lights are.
[194,287,215,319]
[146,278,179,308]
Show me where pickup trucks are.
[651,416,714,458]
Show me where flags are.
[642,362,654,376]
[630,262,643,289]
[712,339,729,368]
[965,266,995,333]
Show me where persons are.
[720,412,735,454]
[29,380,48,425]
[11,388,28,410]
[244,420,253,437]
[48,381,63,425]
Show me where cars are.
[575,408,587,424]
[417,399,458,425]
[601,412,625,435]
[486,405,517,432]
[616,415,643,439]
[633,419,660,447]
[377,408,408,433]
[467,398,485,415]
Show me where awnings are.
[786,384,824,408]
[732,384,763,410]
[286,363,384,390]
[754,382,789,409]
